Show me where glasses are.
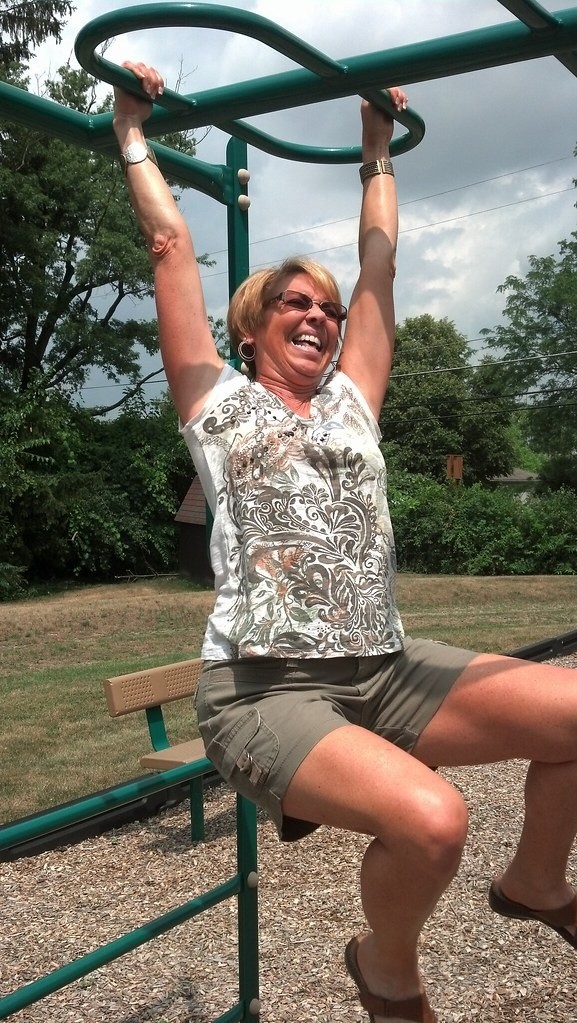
[261,290,348,323]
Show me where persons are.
[112,60,577,1023]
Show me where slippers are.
[345,931,439,1023]
[488,875,577,951]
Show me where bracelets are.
[359,160,395,184]
[119,142,159,177]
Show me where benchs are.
[104,659,227,844]
